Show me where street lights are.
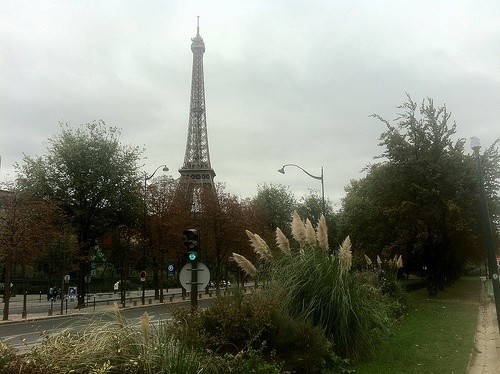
[469,135,500,334]
[279,161,328,250]
[141,164,168,296]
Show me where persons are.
[49,286,58,301]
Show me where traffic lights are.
[182,228,199,266]
